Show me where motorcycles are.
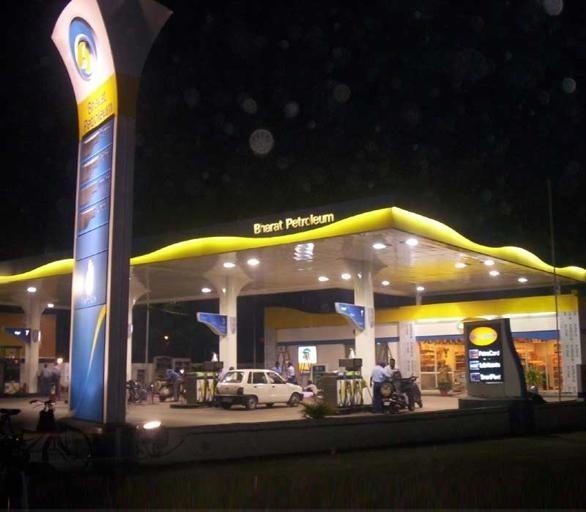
[377,375,423,416]
[125,369,188,403]
[30,397,69,432]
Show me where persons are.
[285,362,297,385]
[39,364,51,397]
[161,368,180,401]
[370,361,394,413]
[303,380,318,397]
[437,362,449,396]
[384,359,401,378]
[299,349,311,372]
[270,361,282,383]
[51,361,62,401]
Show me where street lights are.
[162,334,170,354]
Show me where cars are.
[213,368,304,409]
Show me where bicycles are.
[0,407,91,476]
